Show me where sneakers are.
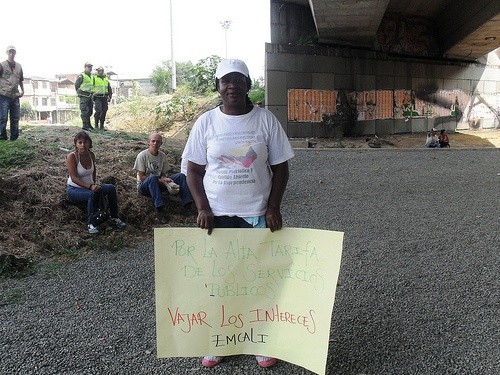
[183,208,195,216]
[107,216,127,229]
[160,212,171,224]
[87,224,99,233]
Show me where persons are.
[94,65,113,131]
[66,131,127,234]
[74,62,95,131]
[133,132,194,222]
[179,58,297,368]
[0,45,25,141]
[438,128,451,148]
[425,128,440,148]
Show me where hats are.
[96,66,104,71]
[84,63,92,67]
[6,45,16,51]
[215,59,249,79]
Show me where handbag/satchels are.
[166,180,180,195]
[90,189,113,227]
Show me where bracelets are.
[89,184,93,189]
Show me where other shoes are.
[100,127,107,131]
[202,356,225,367]
[82,124,94,131]
[256,355,276,367]
